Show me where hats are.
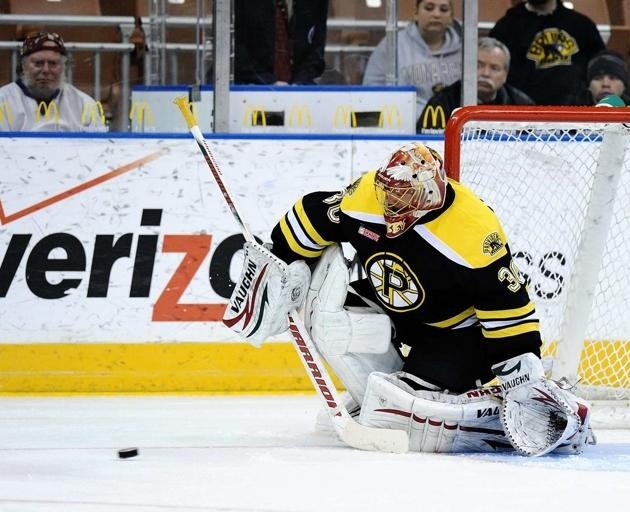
[20,30,68,58]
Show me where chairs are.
[1,1,630,120]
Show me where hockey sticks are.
[173,95,410,452]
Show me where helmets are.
[374,142,448,238]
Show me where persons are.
[221,145,597,458]
[365,0,464,114]
[0,32,108,133]
[210,0,329,86]
[584,50,629,108]
[490,0,605,106]
[417,37,534,135]
